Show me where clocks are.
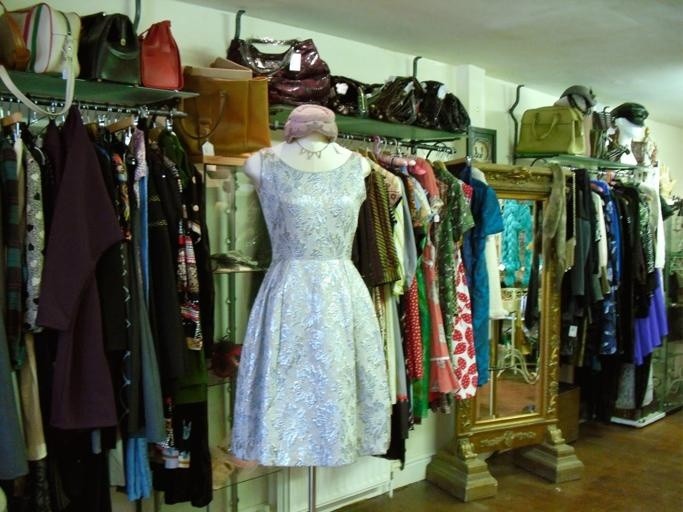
[467,127,497,164]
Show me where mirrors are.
[475,199,543,422]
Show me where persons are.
[611,102,650,165]
[230,102,392,469]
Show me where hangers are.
[0,94,187,137]
[342,133,453,163]
[571,169,644,183]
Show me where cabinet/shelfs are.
[489,288,539,385]
[514,153,683,444]
[200,103,467,511]
[0,71,200,511]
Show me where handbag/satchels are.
[514,106,586,155]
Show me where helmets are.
[611,103,648,124]
[560,86,594,106]
